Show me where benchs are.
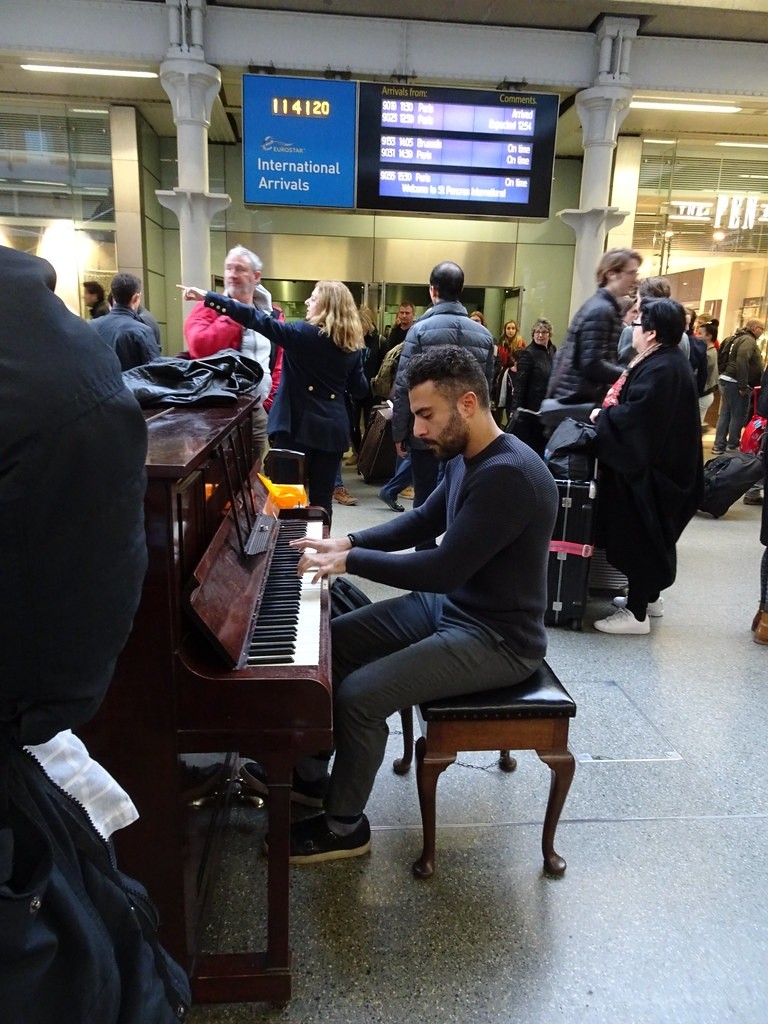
[395,659,576,878]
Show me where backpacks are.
[718,332,746,374]
[689,334,709,397]
[373,342,405,397]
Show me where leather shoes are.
[750,608,768,645]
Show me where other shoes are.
[743,495,764,506]
[345,452,359,465]
[712,446,724,454]
[728,440,741,450]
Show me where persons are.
[0,245,191,1024]
[240,345,559,864]
[174,244,768,646]
[82,273,162,371]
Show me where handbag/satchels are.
[739,414,768,456]
[543,416,602,482]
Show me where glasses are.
[533,330,550,334]
[621,268,638,277]
[631,321,644,328]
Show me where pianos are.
[91,387,340,1009]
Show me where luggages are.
[698,431,768,519]
[357,401,398,484]
[543,418,601,631]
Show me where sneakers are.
[378,491,405,512]
[240,762,332,807]
[333,487,358,504]
[594,606,650,635]
[264,813,372,863]
[613,596,665,617]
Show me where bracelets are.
[348,535,356,548]
